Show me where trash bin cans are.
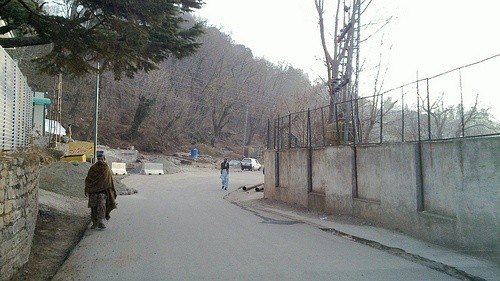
[97,151,104,155]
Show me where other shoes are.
[98,223,106,228]
[91,224,97,229]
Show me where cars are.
[228,159,241,167]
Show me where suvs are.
[240,157,262,172]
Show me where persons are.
[85,155,118,228]
[221,158,230,190]
